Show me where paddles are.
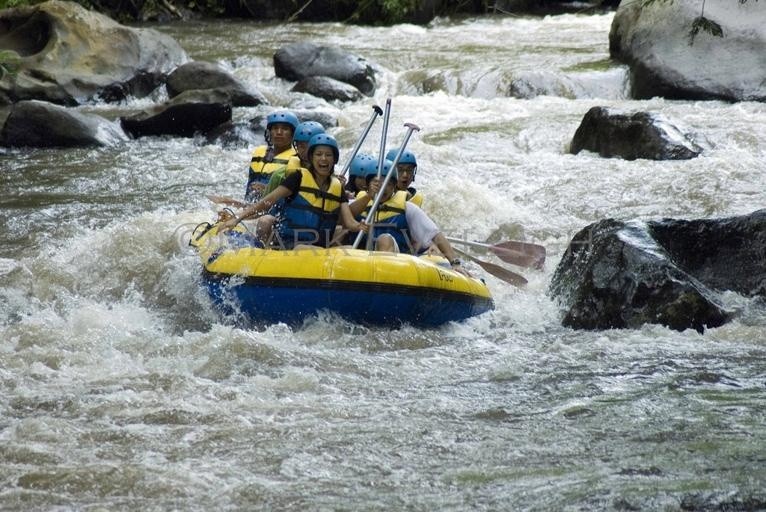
[452,247,528,288]
[208,196,249,208]
[445,237,545,270]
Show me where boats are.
[188,211,496,330]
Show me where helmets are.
[305,132,340,165]
[266,110,300,130]
[348,152,379,180]
[292,121,327,143]
[385,147,418,168]
[364,156,400,185]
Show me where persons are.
[214,111,476,279]
